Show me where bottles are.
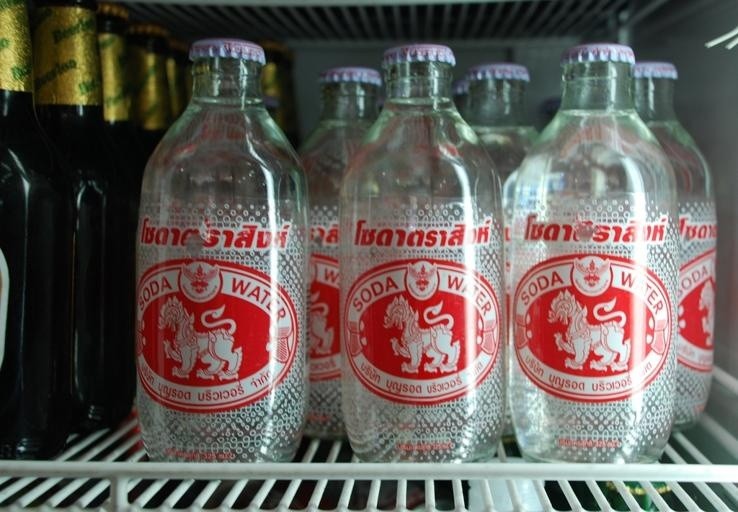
[0,0,716,466]
